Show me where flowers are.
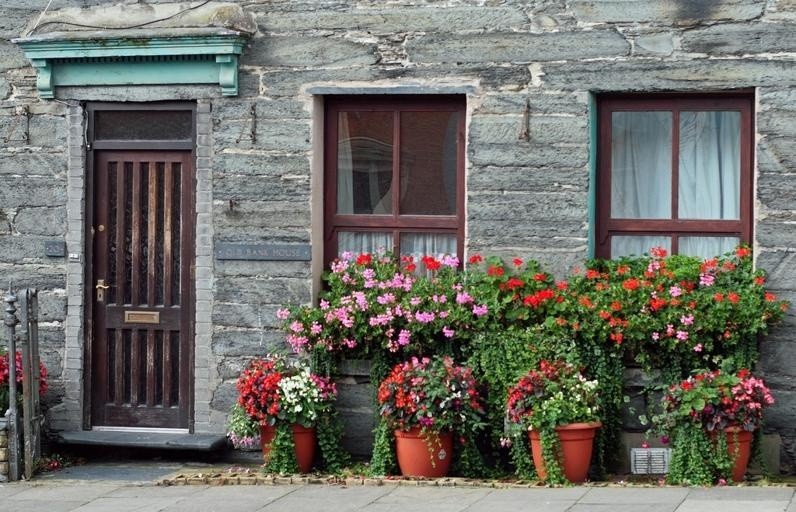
[3,350,49,408]
[226,240,785,422]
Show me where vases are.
[526,424,600,482]
[392,426,452,478]
[706,425,753,479]
[257,423,314,471]
